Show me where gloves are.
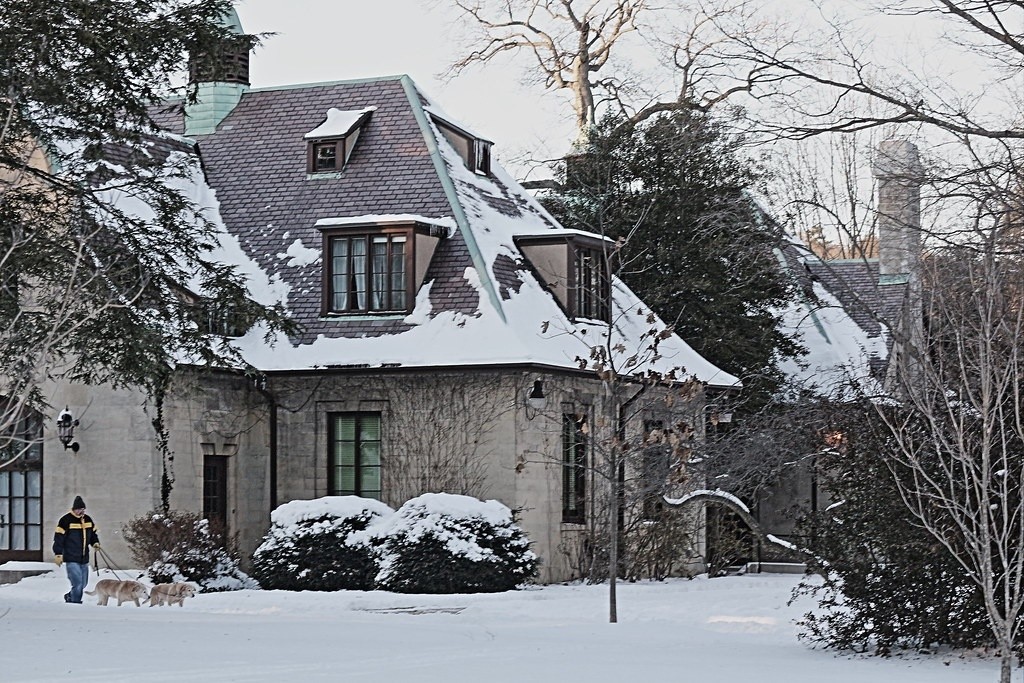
[93,542,101,552]
[56,554,63,567]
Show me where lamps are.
[56,404,79,454]
[523,379,547,420]
[700,391,733,423]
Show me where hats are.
[72,495,86,510]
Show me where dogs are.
[142,583,196,607]
[84,579,151,607]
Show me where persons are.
[53,496,101,604]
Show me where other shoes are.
[65,592,72,603]
